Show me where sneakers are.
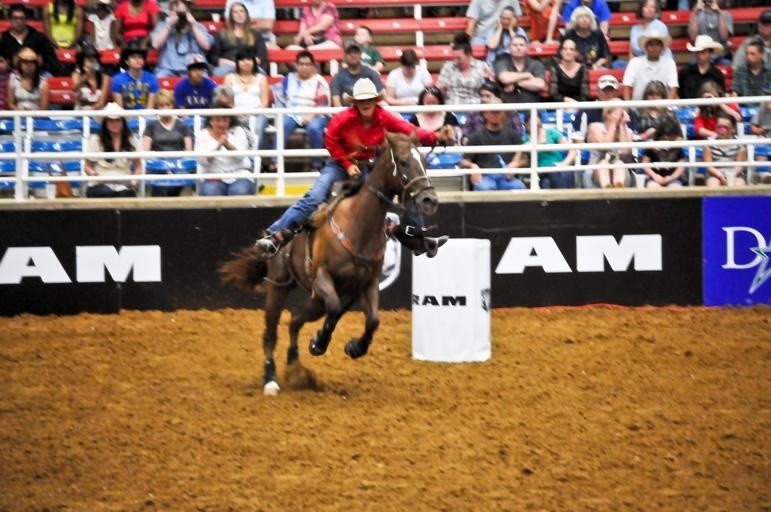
[415,234,449,257]
[255,234,282,258]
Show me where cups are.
[45,184,57,200]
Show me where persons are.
[1,0,770,199]
[252,75,461,261]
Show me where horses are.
[216,127,439,396]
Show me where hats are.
[120,48,148,68]
[598,31,724,90]
[344,42,360,52]
[342,78,386,104]
[93,102,133,124]
[76,45,101,59]
[13,47,42,67]
[187,55,209,71]
